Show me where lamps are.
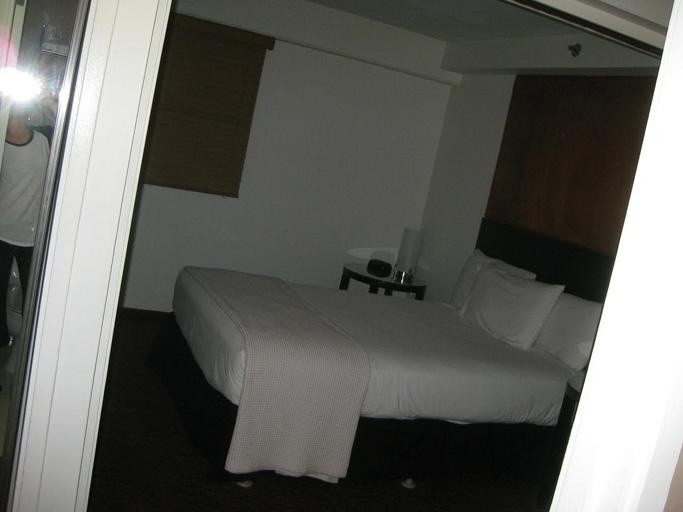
[395,228,425,274]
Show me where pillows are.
[446,247,606,371]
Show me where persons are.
[0,96,58,347]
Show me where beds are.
[148,214,616,489]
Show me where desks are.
[339,262,426,301]
